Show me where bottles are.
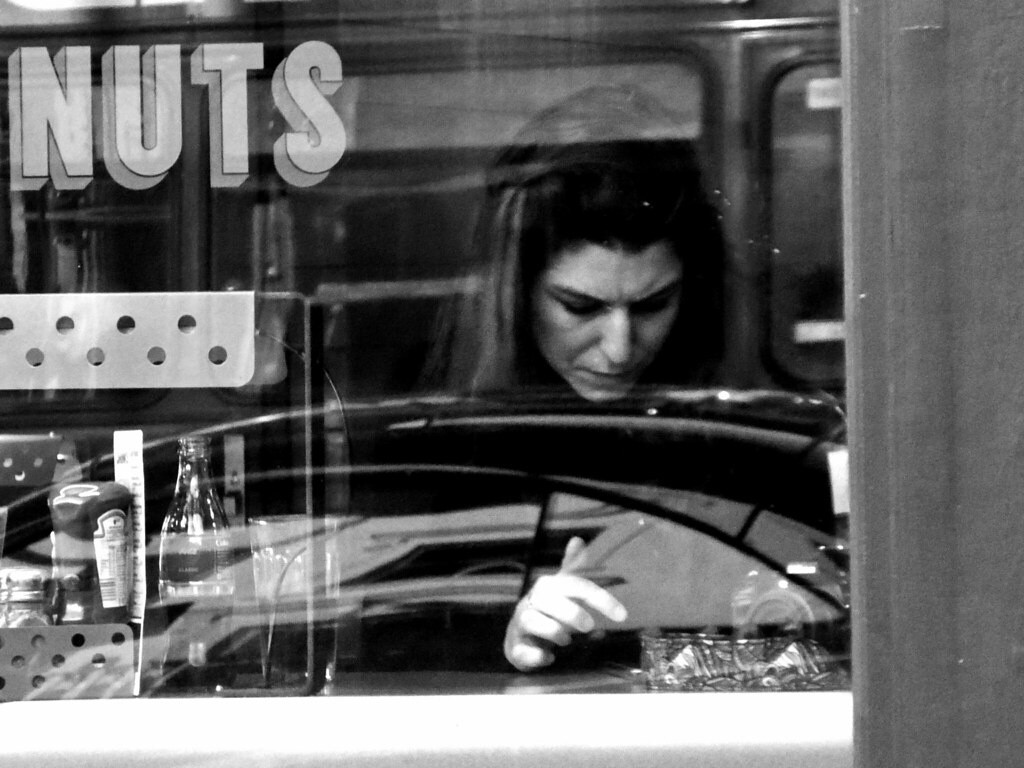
[160,436,235,698]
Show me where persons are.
[412,79,843,675]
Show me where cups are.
[249,515,338,697]
